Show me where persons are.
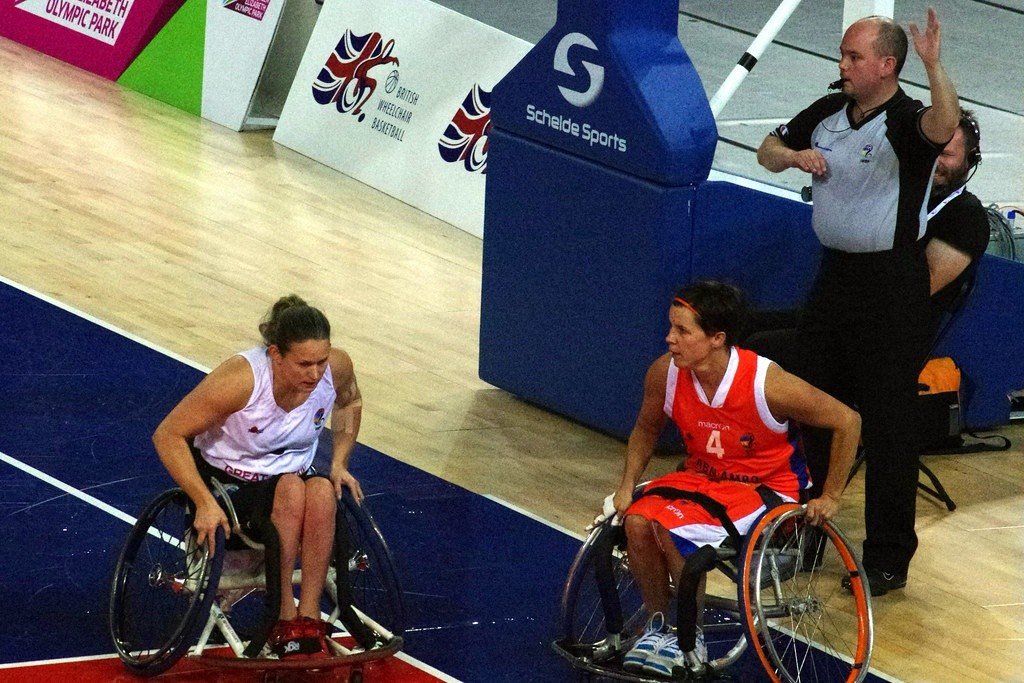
[581,283,863,678]
[152,294,365,658]
[740,104,992,498]
[757,8,960,599]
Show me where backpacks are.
[911,355,1011,455]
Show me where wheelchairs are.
[549,441,874,683]
[108,446,408,683]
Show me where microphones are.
[951,165,979,192]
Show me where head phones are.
[958,114,982,170]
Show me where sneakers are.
[842,565,907,597]
[643,626,708,674]
[622,611,676,672]
[268,613,325,654]
